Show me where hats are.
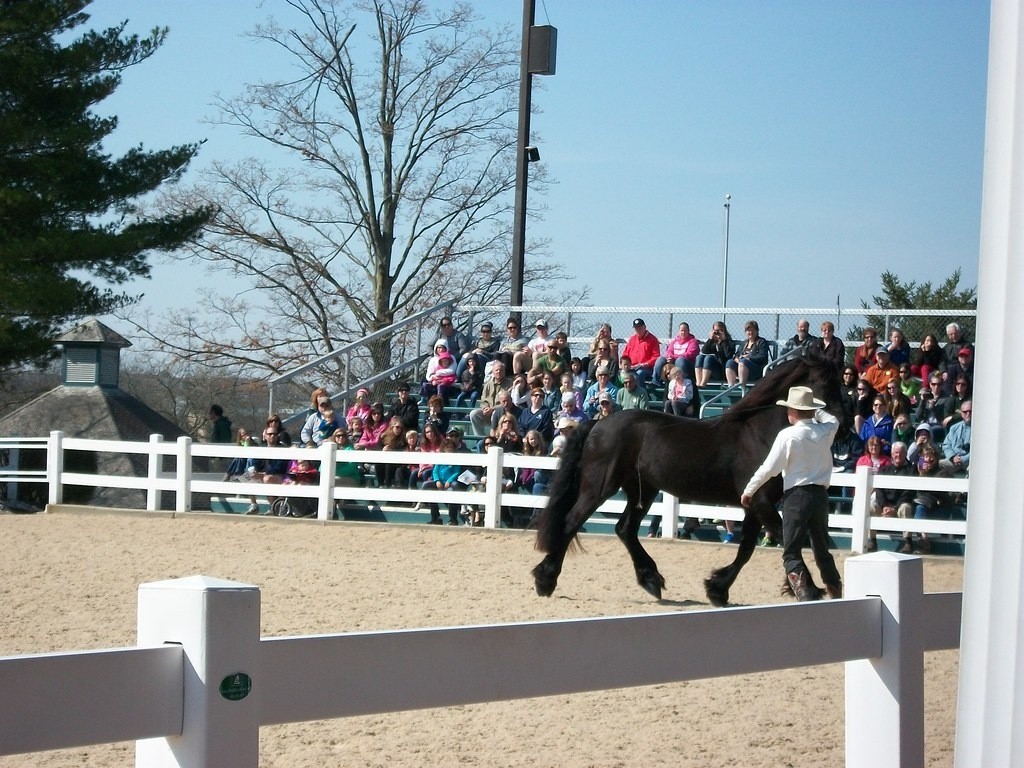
[536,318,547,328]
[448,426,463,440]
[482,322,493,328]
[318,382,410,414]
[959,349,972,357]
[632,319,645,327]
[776,386,826,411]
[598,392,612,405]
[554,417,578,430]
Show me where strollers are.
[271,471,319,517]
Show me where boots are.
[828,581,842,599]
[788,571,812,602]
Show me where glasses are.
[481,326,608,376]
[449,434,460,438]
[821,329,833,332]
[441,323,450,327]
[503,394,544,424]
[268,419,403,439]
[844,336,972,414]
[484,443,490,446]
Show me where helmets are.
[876,346,888,354]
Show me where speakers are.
[529,24,557,75]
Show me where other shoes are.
[245,505,274,515]
[426,519,459,526]
[913,537,931,554]
[867,538,878,551]
[899,537,911,554]
[954,493,967,504]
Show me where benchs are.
[203,333,974,557]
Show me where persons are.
[741,386,842,601]
[209,317,975,554]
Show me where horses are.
[530,355,849,605]
